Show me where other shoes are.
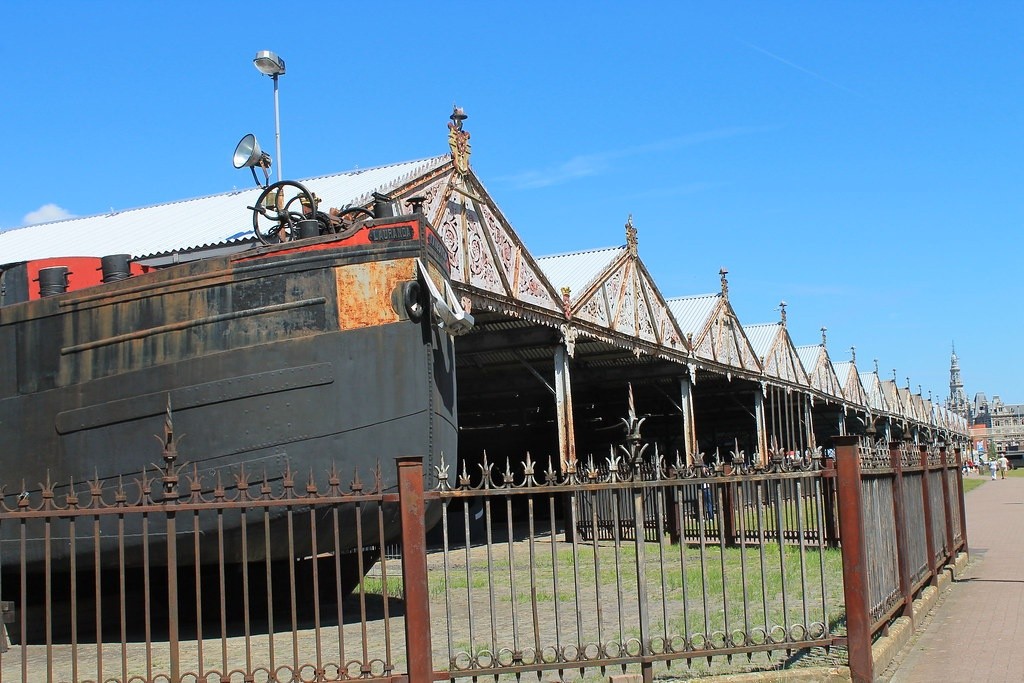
[992,478,996,480]
[1002,477,1004,479]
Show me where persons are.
[988,453,1009,480]
[962,457,984,475]
[697,464,713,519]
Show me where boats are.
[1,180,474,611]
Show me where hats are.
[1000,453,1004,456]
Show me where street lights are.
[251,50,286,244]
